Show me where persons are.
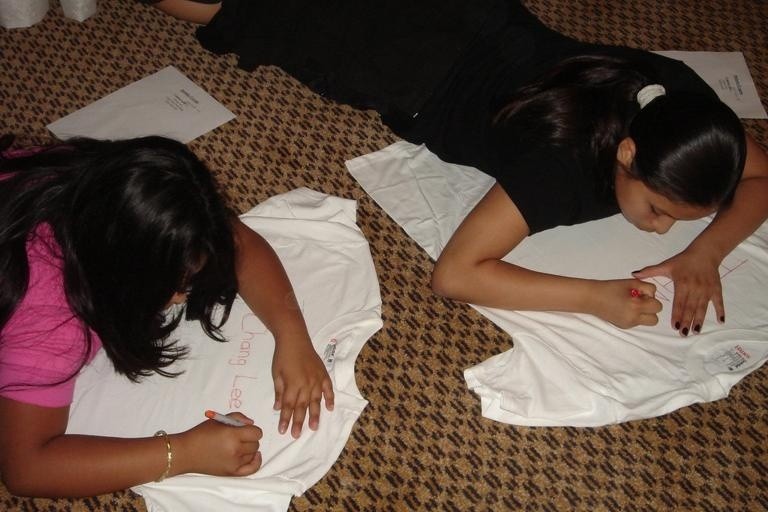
[0,127,337,501]
[149,2,768,337]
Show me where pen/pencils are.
[205,410,247,427]
[631,288,640,297]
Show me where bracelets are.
[152,429,172,483]
